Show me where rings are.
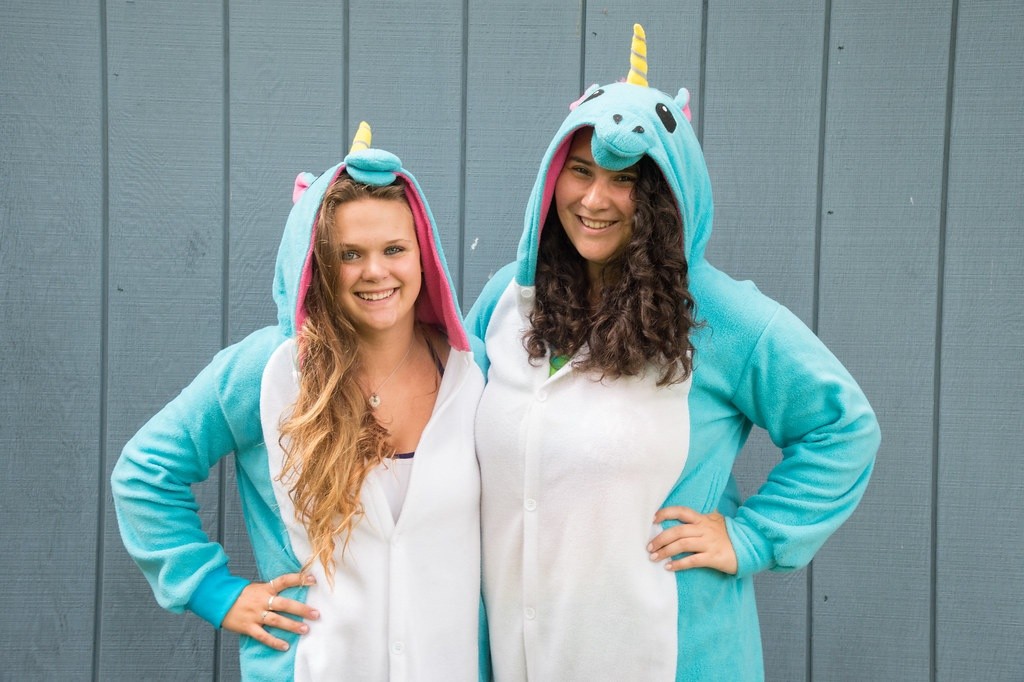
[262,611,269,622]
[270,580,277,594]
[269,596,276,611]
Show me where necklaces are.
[353,331,416,407]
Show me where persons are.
[468,22,883,682]
[110,121,489,682]
